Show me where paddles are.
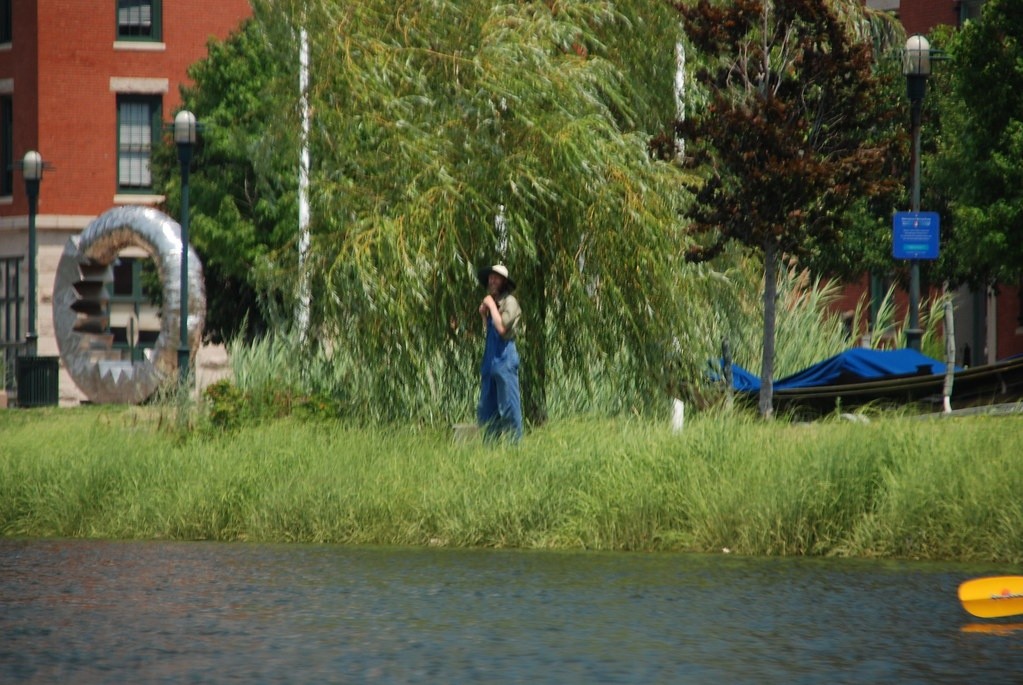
[957,575,1023,618]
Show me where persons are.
[476,264,524,444]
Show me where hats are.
[477,264,517,292]
